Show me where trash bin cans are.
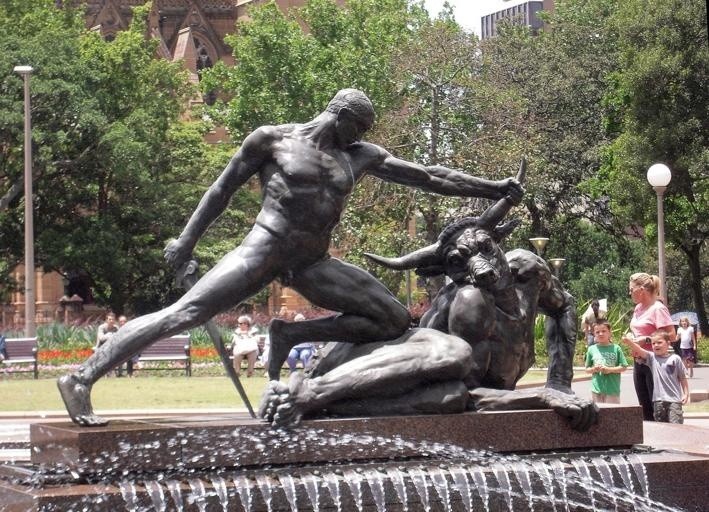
[672,324,697,363]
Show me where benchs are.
[0,338,40,378]
[132,336,192,374]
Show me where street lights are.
[546,257,566,278]
[528,234,549,258]
[13,65,36,338]
[647,163,671,309]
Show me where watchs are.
[645,336,652,344]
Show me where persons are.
[225,315,258,377]
[286,313,314,372]
[668,317,697,376]
[584,319,628,402]
[55,89,524,427]
[96,312,119,379]
[619,330,689,423]
[581,299,605,354]
[261,308,288,378]
[0,334,6,361]
[624,273,678,419]
[118,315,136,377]
